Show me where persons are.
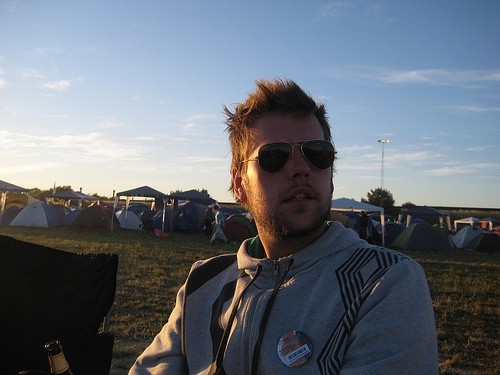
[209,205,231,247]
[359,210,368,237]
[127,78,441,375]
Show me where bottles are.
[44,339,73,375]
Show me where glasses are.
[240,140,337,173]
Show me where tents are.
[0,180,34,192]
[368,216,430,242]
[393,222,447,250]
[451,225,484,248]
[219,207,258,239]
[46,190,97,206]
[454,217,480,232]
[110,186,218,234]
[330,197,385,247]
[0,201,117,228]
[468,232,500,252]
